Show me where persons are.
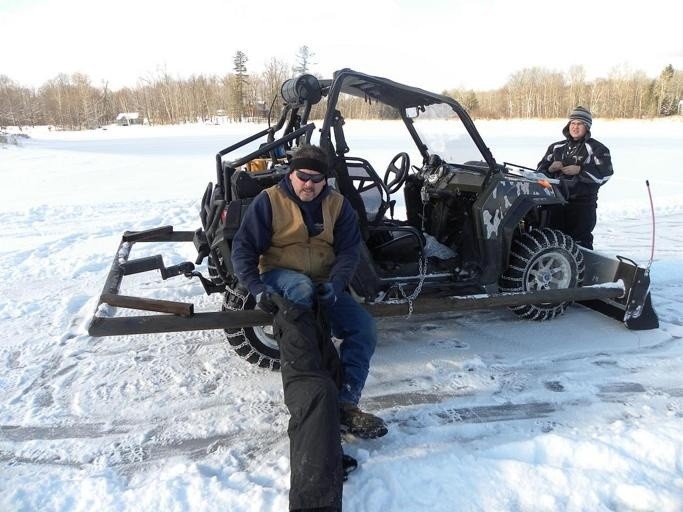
[230,144,388,474]
[536,106,614,251]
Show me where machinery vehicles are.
[88,70,659,370]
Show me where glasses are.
[295,169,325,183]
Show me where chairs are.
[344,155,427,276]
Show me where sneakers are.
[339,402,388,439]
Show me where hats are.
[289,144,327,183]
[562,106,592,143]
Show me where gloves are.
[250,282,278,316]
[317,282,338,309]
